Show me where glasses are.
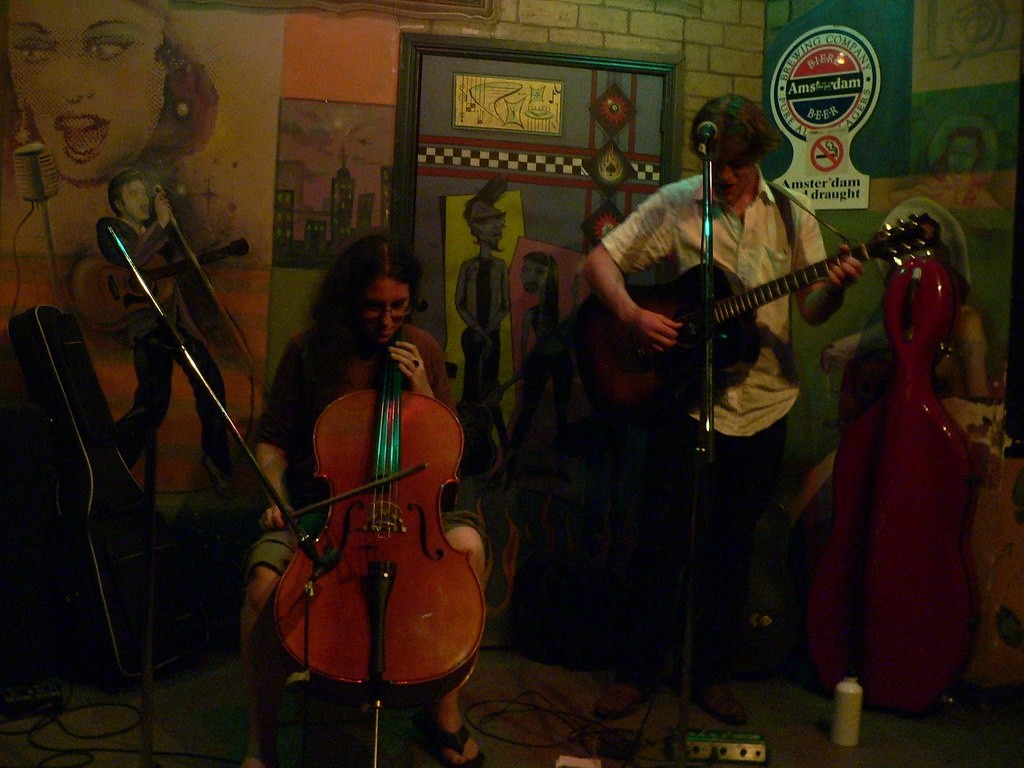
[363,303,412,316]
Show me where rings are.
[412,359,420,367]
[264,522,268,528]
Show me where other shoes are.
[593,681,656,716]
[699,684,744,724]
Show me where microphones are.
[698,121,718,142]
[308,547,340,584]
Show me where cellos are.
[270,291,488,768]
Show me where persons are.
[241,232,486,768]
[583,96,864,718]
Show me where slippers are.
[422,710,484,768]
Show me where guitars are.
[572,213,944,417]
